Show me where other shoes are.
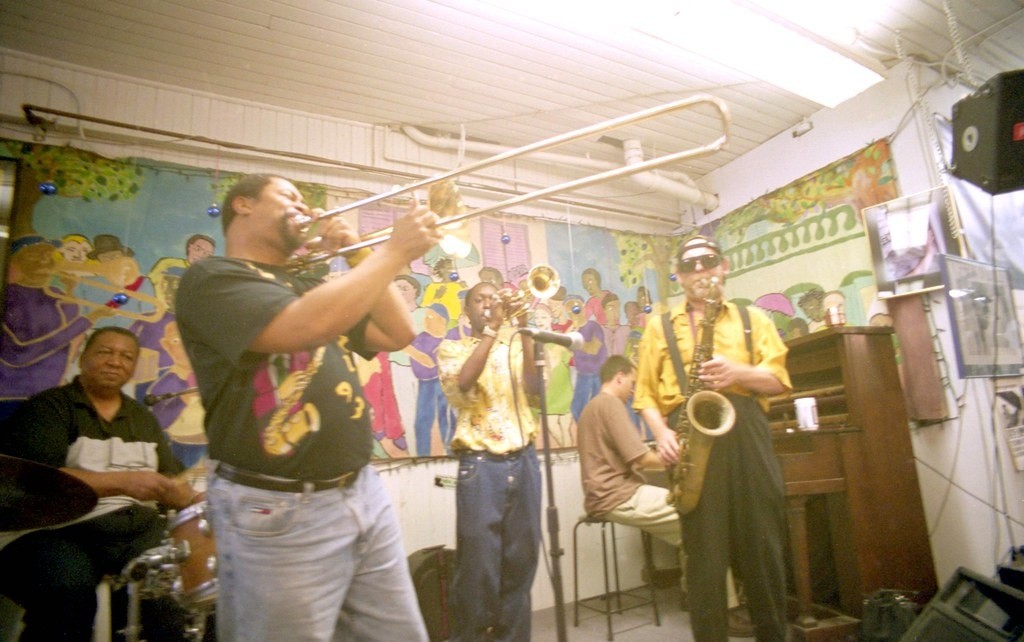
[681,592,690,611]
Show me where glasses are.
[678,253,724,274]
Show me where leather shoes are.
[727,610,756,637]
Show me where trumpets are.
[483,262,562,316]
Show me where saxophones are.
[667,275,735,517]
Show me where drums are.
[168,501,220,609]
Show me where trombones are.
[288,89,733,266]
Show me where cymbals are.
[0,455,98,532]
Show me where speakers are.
[900,566,1024,642]
[951,69,1024,196]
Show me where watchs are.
[482,326,497,338]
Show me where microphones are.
[144,392,173,408]
[520,327,584,351]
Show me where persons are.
[634,231,792,642]
[575,355,757,638]
[1,328,209,642]
[171,175,443,642]
[439,280,550,642]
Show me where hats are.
[678,236,722,253]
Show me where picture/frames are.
[862,186,968,301]
[937,252,1024,379]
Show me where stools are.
[573,516,662,642]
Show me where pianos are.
[641,325,941,626]
